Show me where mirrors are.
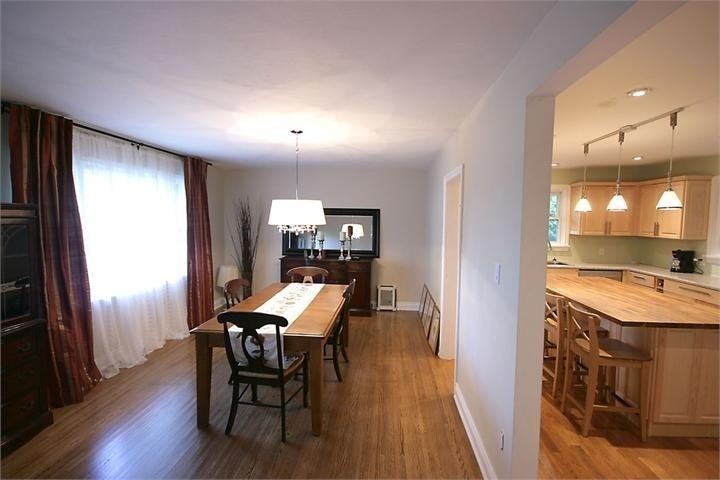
[282,208,380,258]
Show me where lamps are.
[341,224,364,240]
[267,130,327,236]
[574,107,684,212]
[217,266,242,311]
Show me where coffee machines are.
[670,250,694,273]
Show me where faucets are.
[548,240,552,252]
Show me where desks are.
[189,283,349,436]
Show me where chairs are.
[546,268,653,440]
[286,266,328,283]
[293,277,356,382]
[224,279,278,388]
[217,311,308,443]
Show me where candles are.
[348,226,353,236]
[340,232,346,241]
[311,226,317,235]
[317,231,324,241]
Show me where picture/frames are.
[418,284,440,354]
[376,285,397,312]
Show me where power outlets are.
[500,431,504,449]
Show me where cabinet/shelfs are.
[0,202,53,460]
[570,176,712,240]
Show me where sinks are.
[547,261,569,265]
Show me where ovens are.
[578,270,622,282]
